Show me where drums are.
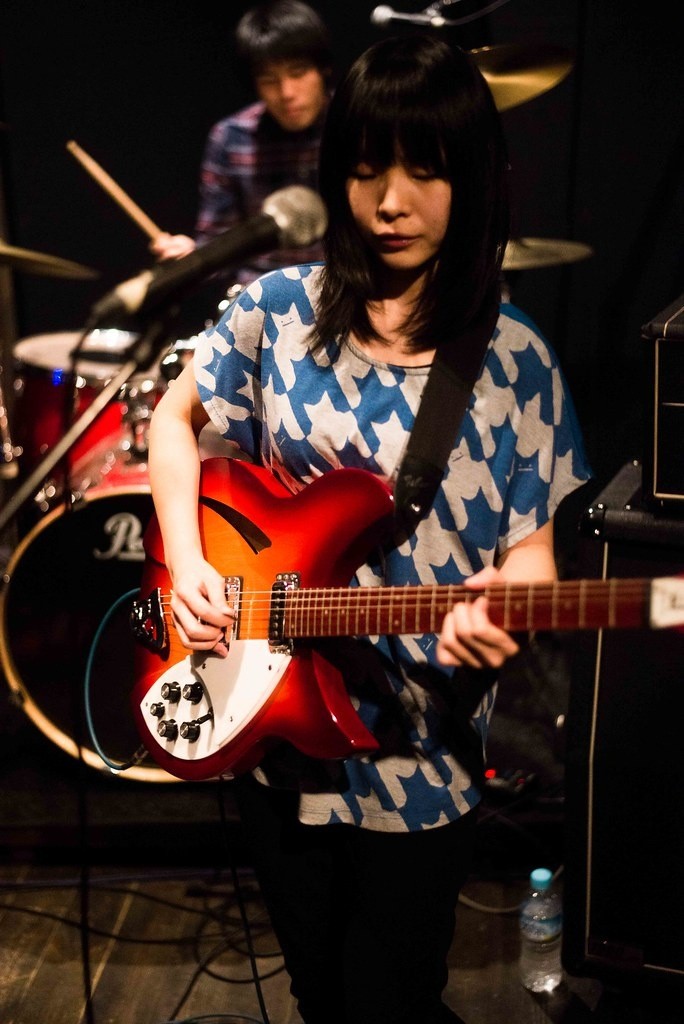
[0,328,235,784]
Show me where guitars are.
[0,456,684,784]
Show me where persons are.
[194,0,339,287]
[146,32,592,1024]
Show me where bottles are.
[518,868,563,993]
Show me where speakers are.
[561,456,684,994]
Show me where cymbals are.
[0,237,99,280]
[469,44,576,114]
[496,238,596,271]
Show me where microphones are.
[90,184,330,331]
